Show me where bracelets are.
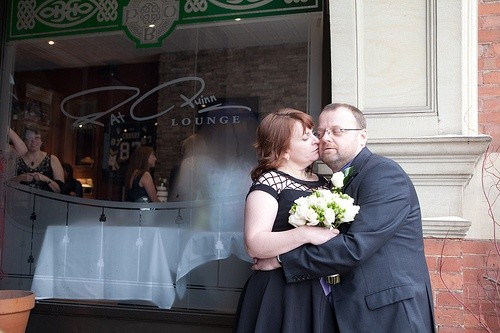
[276,255,283,266]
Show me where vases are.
[0,290,35,333]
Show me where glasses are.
[313,125,363,139]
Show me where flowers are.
[289,170,361,283]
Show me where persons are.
[0,121,160,203]
[232,107,340,333]
[250,102,437,333]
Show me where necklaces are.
[28,152,41,166]
